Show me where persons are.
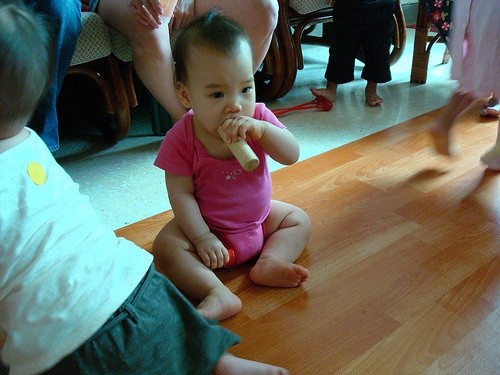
[426,1,500,172]
[0,1,309,375]
[310,0,396,107]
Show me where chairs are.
[409,0,442,84]
[255,0,406,102]
[64,12,130,142]
[109,25,184,137]
